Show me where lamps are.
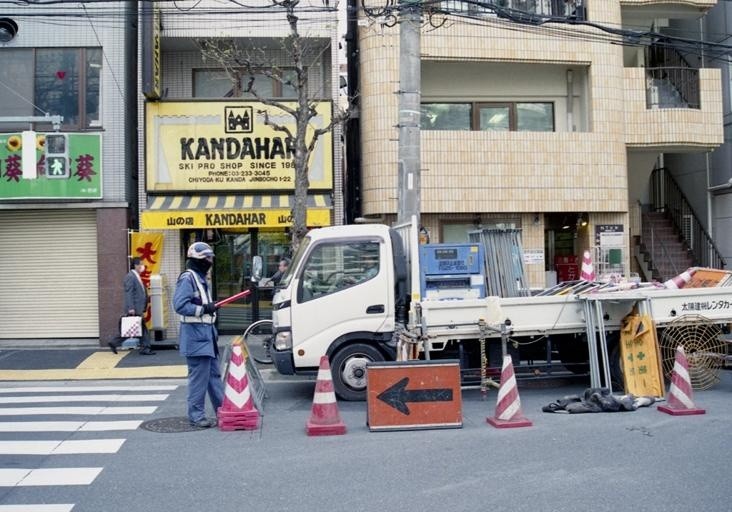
[0,18,19,45]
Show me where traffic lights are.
[45,133,70,179]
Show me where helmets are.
[186,241,215,259]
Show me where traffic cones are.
[305,355,346,436]
[217,344,260,431]
[487,355,532,428]
[657,345,705,416]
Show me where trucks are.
[249,215,731,401]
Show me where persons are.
[108,257,157,355]
[173,242,226,428]
[270,257,291,281]
[342,252,378,284]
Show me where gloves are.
[203,301,221,314]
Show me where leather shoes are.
[189,416,217,427]
[108,342,118,354]
[139,350,156,355]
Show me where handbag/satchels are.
[121,316,142,337]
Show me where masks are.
[138,265,146,272]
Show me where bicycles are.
[242,319,274,364]
